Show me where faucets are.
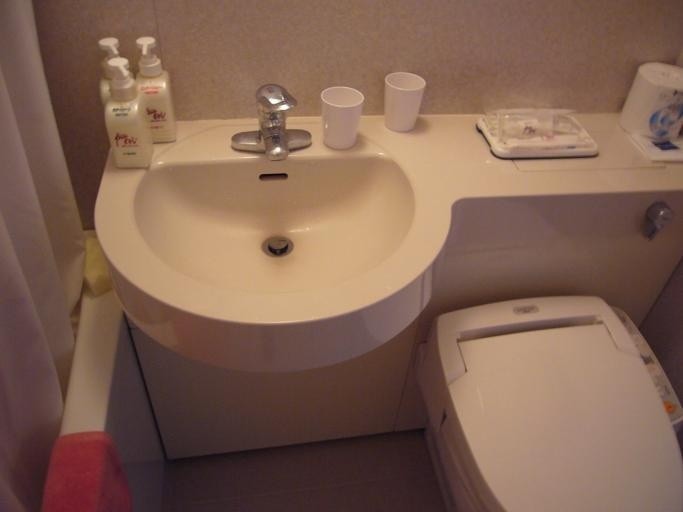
[228,84,311,164]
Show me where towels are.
[40,431,134,512]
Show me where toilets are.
[412,291,683,512]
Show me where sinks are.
[87,148,456,378]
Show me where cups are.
[319,86,364,153]
[384,70,426,135]
